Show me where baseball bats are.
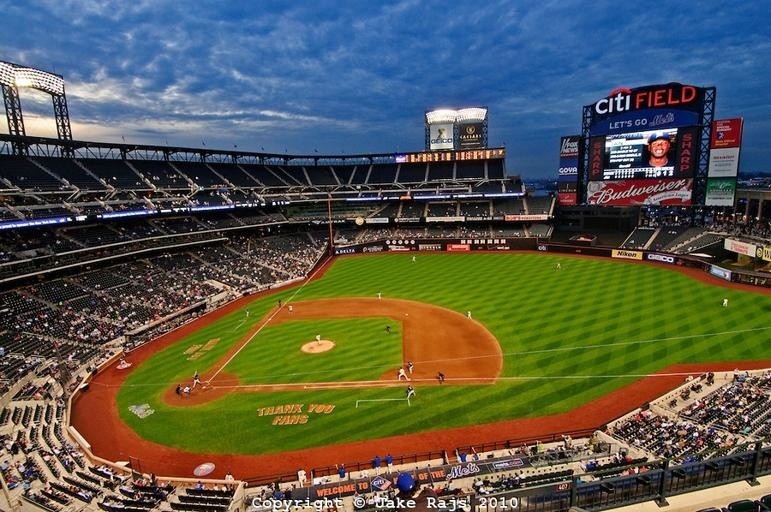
[193,370,197,378]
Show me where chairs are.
[502,392,771,512]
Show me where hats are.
[397,473,414,491]
[648,131,672,144]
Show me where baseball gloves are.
[191,388,193,390]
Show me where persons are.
[632,131,678,177]
[0,172,770,511]
